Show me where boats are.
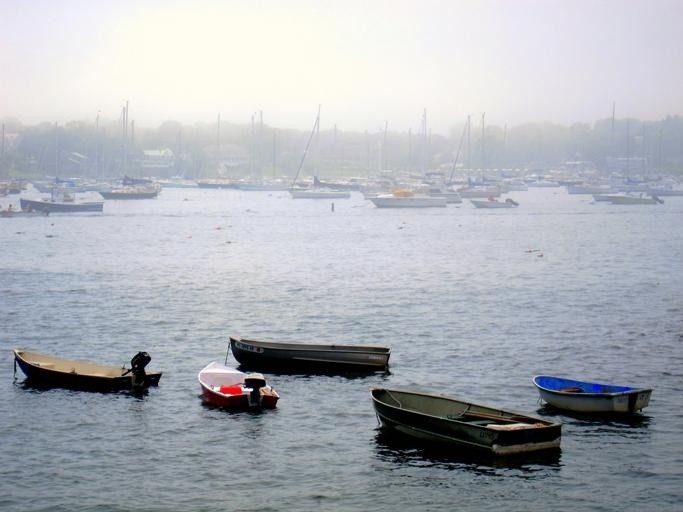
[368,386,562,460]
[197,360,280,414]
[531,373,653,418]
[469,198,520,208]
[228,334,391,376]
[0,206,51,217]
[368,189,447,209]
[11,345,162,394]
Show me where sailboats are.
[0,99,163,199]
[287,103,351,200]
[18,121,106,212]
[323,108,561,199]
[158,109,293,192]
[566,99,682,205]
